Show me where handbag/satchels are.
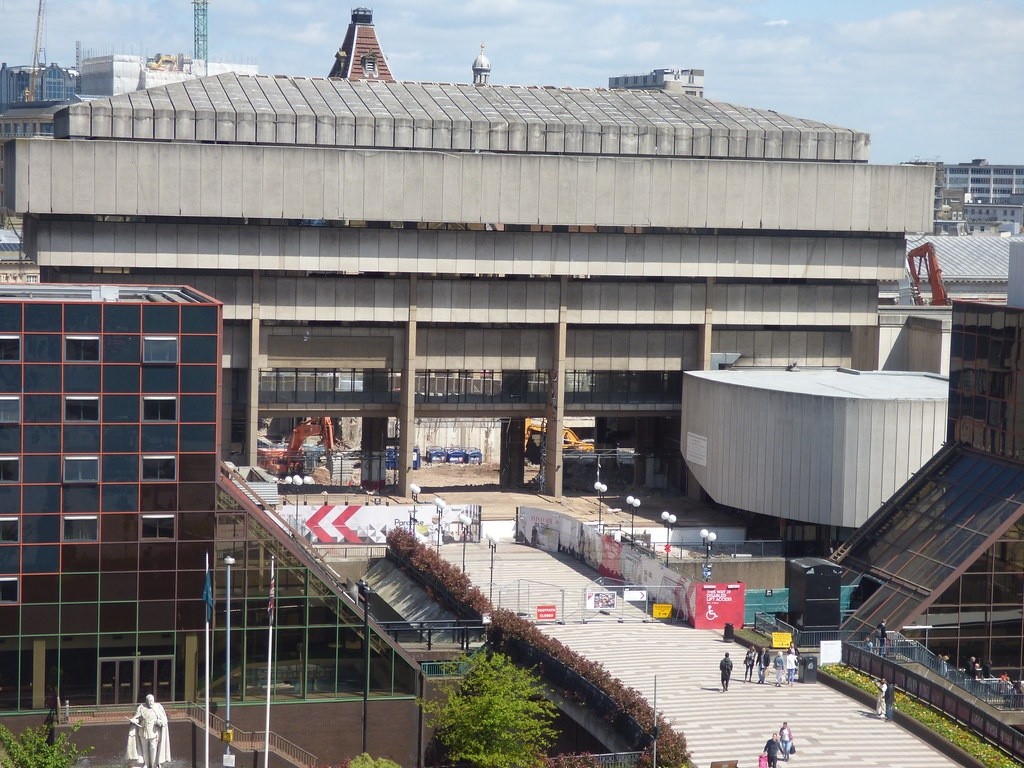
[790,742,796,754]
[759,754,769,768]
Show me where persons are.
[764,733,785,768]
[938,651,1024,711]
[779,722,793,761]
[863,634,872,653]
[125,694,172,768]
[876,619,887,658]
[875,678,894,722]
[720,642,801,692]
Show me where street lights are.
[626,496,641,550]
[662,511,677,567]
[594,482,607,533]
[700,529,716,583]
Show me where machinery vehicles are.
[265,416,334,479]
[907,243,951,306]
[525,418,594,454]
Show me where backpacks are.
[722,663,730,679]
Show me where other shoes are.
[784,755,789,762]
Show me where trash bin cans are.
[723,622,736,643]
[798,655,817,683]
